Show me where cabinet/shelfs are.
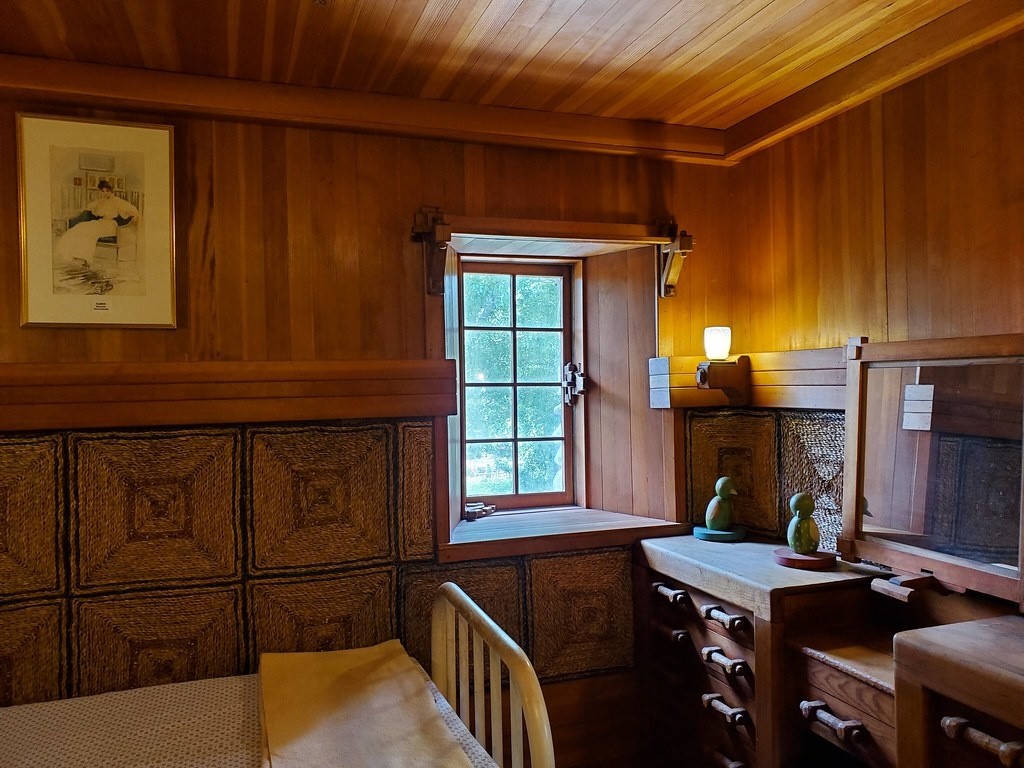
[898,614,1024,768]
[800,623,899,767]
[639,533,889,768]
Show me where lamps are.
[703,327,732,361]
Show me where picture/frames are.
[12,108,182,330]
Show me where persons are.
[57,179,139,265]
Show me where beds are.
[3,653,505,767]
